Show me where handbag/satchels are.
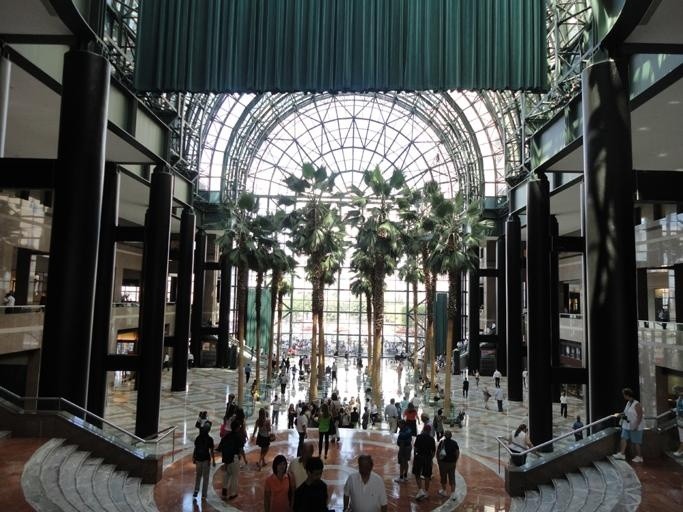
[269,433,276,442]
[328,417,336,435]
[438,440,447,460]
[332,404,338,417]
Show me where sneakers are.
[439,489,446,496]
[193,491,237,500]
[451,493,456,500]
[424,492,429,497]
[394,478,404,482]
[415,489,425,499]
[404,478,408,481]
[238,459,267,471]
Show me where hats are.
[442,431,452,437]
[424,425,431,431]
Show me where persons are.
[462,377,469,398]
[521,368,528,387]
[161,351,169,372]
[571,414,584,442]
[492,369,502,388]
[493,383,505,412]
[473,368,480,388]
[507,423,535,466]
[1,292,15,313]
[610,387,645,463]
[187,351,194,369]
[189,335,460,511]
[559,392,567,417]
[480,386,491,410]
[671,385,683,457]
